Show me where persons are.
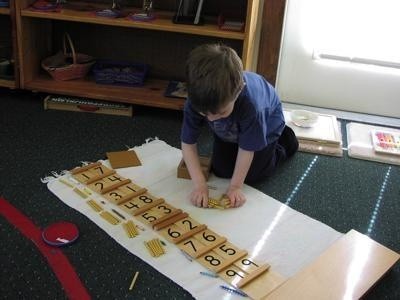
[180,42,298,208]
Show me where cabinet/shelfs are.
[0,0,266,112]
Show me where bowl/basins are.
[291,109,319,129]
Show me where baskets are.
[40,28,99,81]
[91,58,151,88]
